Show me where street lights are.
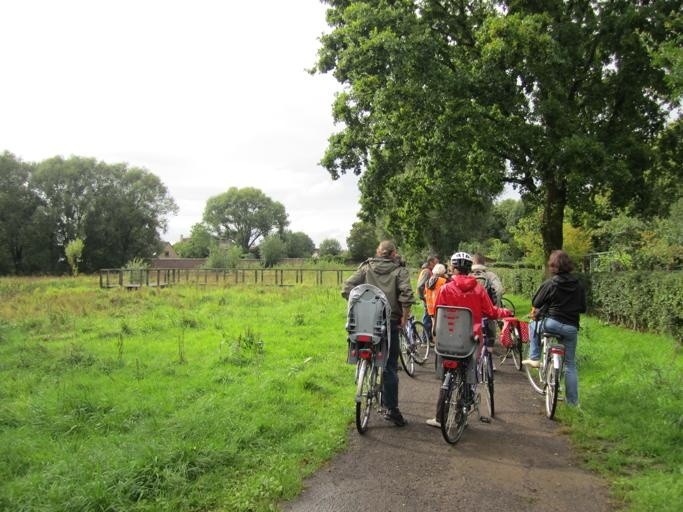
[535,302,548,319]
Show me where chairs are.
[426,418,441,428]
[421,342,435,348]
[384,408,405,426]
[522,359,540,368]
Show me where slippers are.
[473,271,496,306]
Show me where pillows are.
[451,252,472,269]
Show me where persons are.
[471,251,506,372]
[340,239,417,427]
[425,250,513,428]
[423,263,448,337]
[520,248,589,409]
[415,252,440,348]
